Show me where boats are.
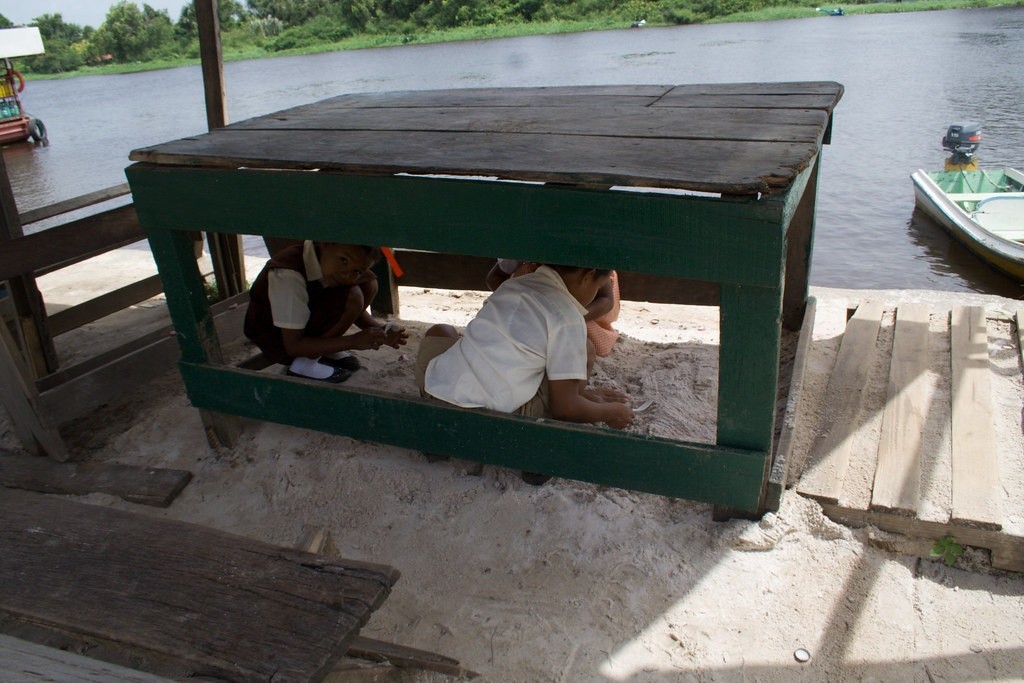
[909,121,1024,278]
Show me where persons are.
[414,263,636,486]
[243,239,409,384]
[485,258,621,396]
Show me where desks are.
[125,81,845,524]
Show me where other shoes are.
[522,470,552,485]
[319,355,360,370]
[287,364,351,383]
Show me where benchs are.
[990,230,1024,241]
[0,486,400,682]
[948,191,1024,202]
